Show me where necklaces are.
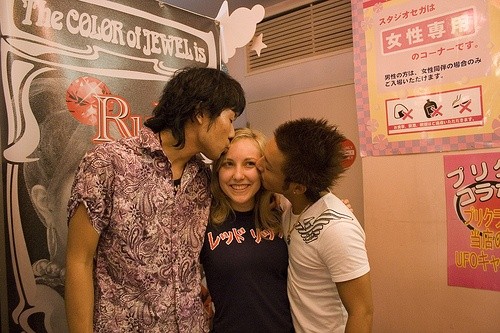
[156,129,188,188]
[286,202,314,245]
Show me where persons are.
[63,67,246,331]
[199,123,293,333]
[254,116,373,333]
